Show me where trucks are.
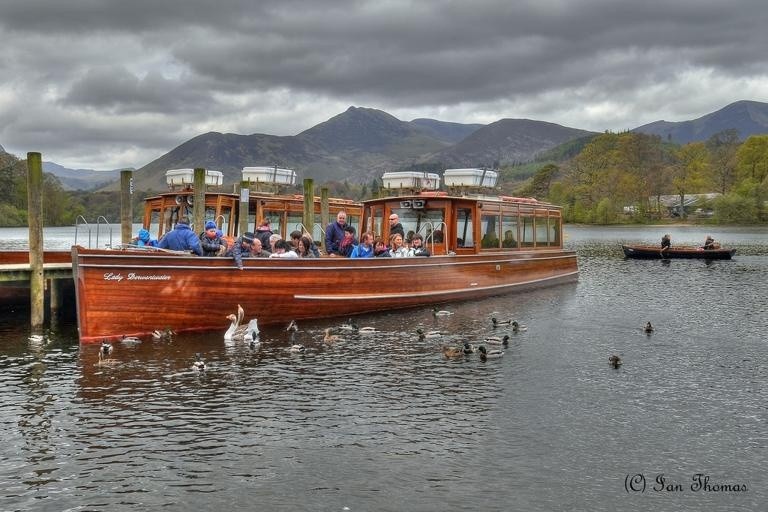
[622,206,646,215]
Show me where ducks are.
[151,327,176,338]
[98,337,114,359]
[489,317,528,332]
[121,334,143,346]
[223,303,260,341]
[323,318,359,348]
[607,353,622,369]
[478,346,505,359]
[642,321,656,333]
[431,306,455,317]
[440,345,464,357]
[483,335,510,345]
[416,328,445,339]
[286,319,300,334]
[462,343,477,354]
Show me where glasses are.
[388,218,396,221]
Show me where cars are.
[696,207,714,218]
[670,210,689,218]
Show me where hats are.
[139,229,150,242]
[205,221,216,229]
[243,232,255,242]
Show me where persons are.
[480,228,500,248]
[197,220,228,258]
[324,210,444,259]
[269,233,282,248]
[249,237,272,259]
[699,234,714,251]
[156,216,204,257]
[501,229,517,248]
[298,236,317,258]
[660,234,671,248]
[254,216,272,252]
[224,231,254,271]
[303,232,321,258]
[289,230,302,248]
[268,239,299,259]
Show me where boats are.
[621,244,737,259]
[70,191,580,345]
[0,188,366,309]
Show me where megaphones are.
[399,201,411,209]
[175,196,184,204]
[412,200,425,209]
[186,196,193,205]
